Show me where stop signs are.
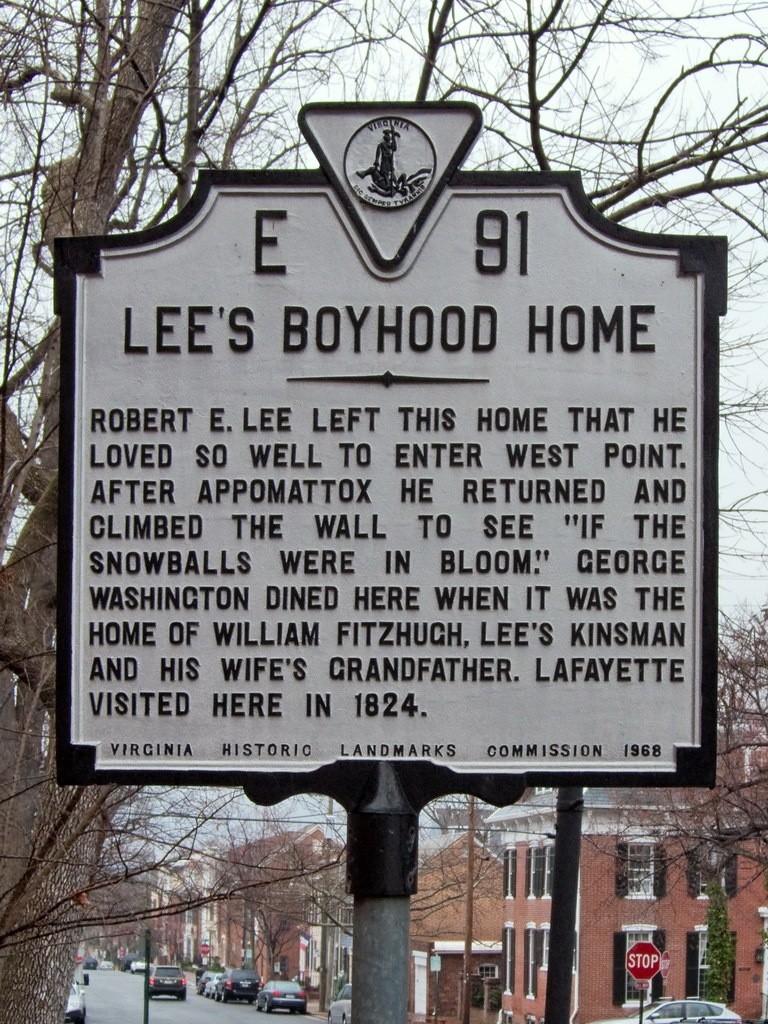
[625,940,662,982]
[660,951,672,979]
[199,943,210,955]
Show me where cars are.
[326,982,352,1024]
[585,997,741,1024]
[64,973,89,1024]
[257,980,308,1014]
[76,954,114,970]
[196,970,224,999]
[128,958,153,974]
[115,953,139,971]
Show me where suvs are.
[214,968,266,1005]
[149,964,187,1001]
